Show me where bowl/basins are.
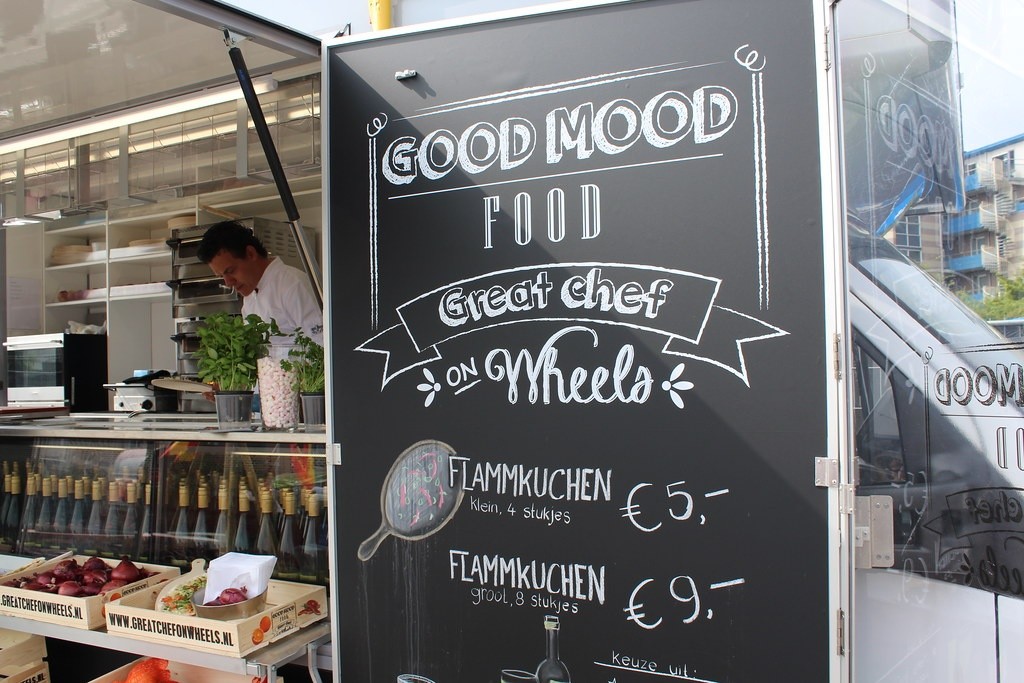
[191,585,270,622]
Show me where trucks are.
[0,0,1024,683]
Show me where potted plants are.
[279,327,327,428]
[194,310,275,433]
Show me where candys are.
[257,357,301,429]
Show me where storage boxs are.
[0,549,328,656]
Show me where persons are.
[195,220,324,404]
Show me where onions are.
[3,554,154,596]
[202,586,248,605]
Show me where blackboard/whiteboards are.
[322,0,851,682]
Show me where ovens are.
[3,330,109,413]
[166,217,316,376]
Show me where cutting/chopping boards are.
[151,378,214,393]
[154,559,206,617]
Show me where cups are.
[369,0,392,31]
[299,395,325,433]
[257,343,302,433]
[92,242,105,252]
[212,395,254,431]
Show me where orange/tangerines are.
[252,616,271,645]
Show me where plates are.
[128,215,197,247]
[49,243,91,263]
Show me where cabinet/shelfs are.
[38,217,200,384]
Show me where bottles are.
[1,458,330,596]
